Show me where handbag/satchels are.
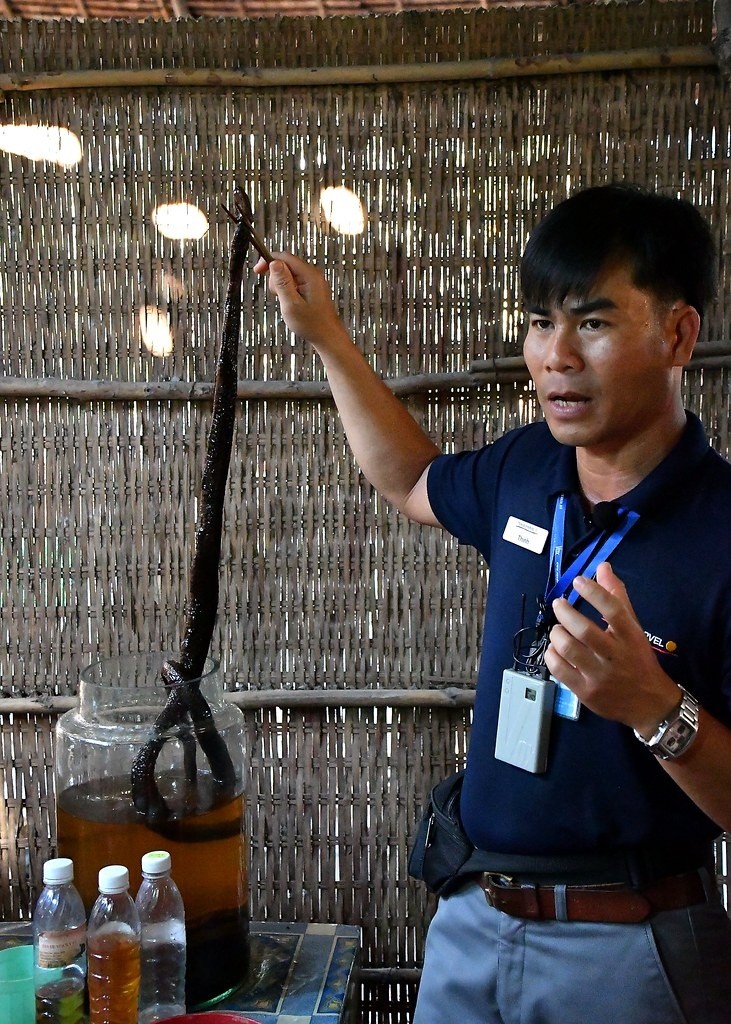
[407,767,715,900]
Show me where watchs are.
[633,684,699,762]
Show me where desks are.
[0,921,362,1024]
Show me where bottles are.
[86,864,141,1024]
[53,652,253,1014]
[32,858,88,1024]
[135,850,187,1024]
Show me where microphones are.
[593,500,620,530]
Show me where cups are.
[0,945,63,1024]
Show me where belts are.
[477,869,721,923]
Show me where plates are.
[150,1013,261,1024]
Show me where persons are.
[253,185,731,1024]
